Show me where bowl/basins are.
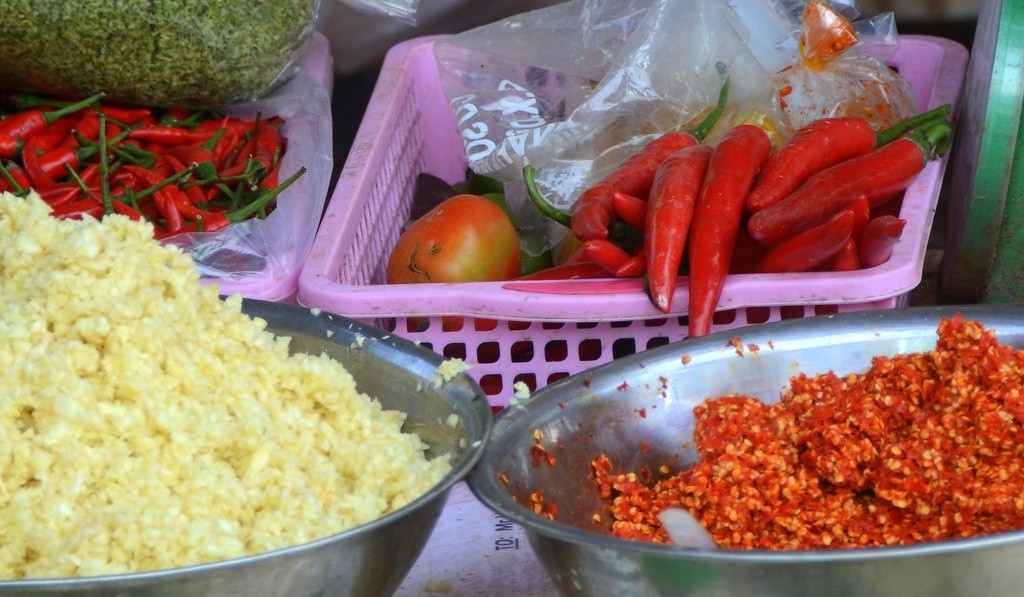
[465,307,1024,597]
[0,298,492,597]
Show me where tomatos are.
[385,193,520,325]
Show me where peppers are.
[416,60,952,414]
[0,84,307,247]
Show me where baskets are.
[197,30,336,301]
[296,34,970,417]
